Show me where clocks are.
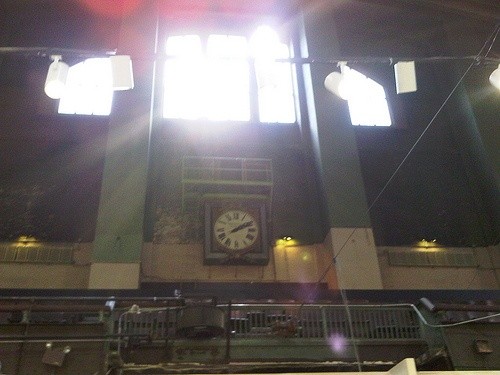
[203,199,269,265]
